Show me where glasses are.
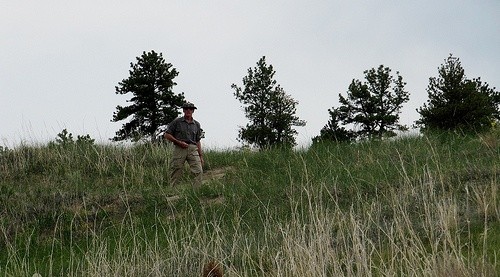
[185,108,193,110]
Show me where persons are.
[165,103,205,188]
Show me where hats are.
[181,103,197,109]
